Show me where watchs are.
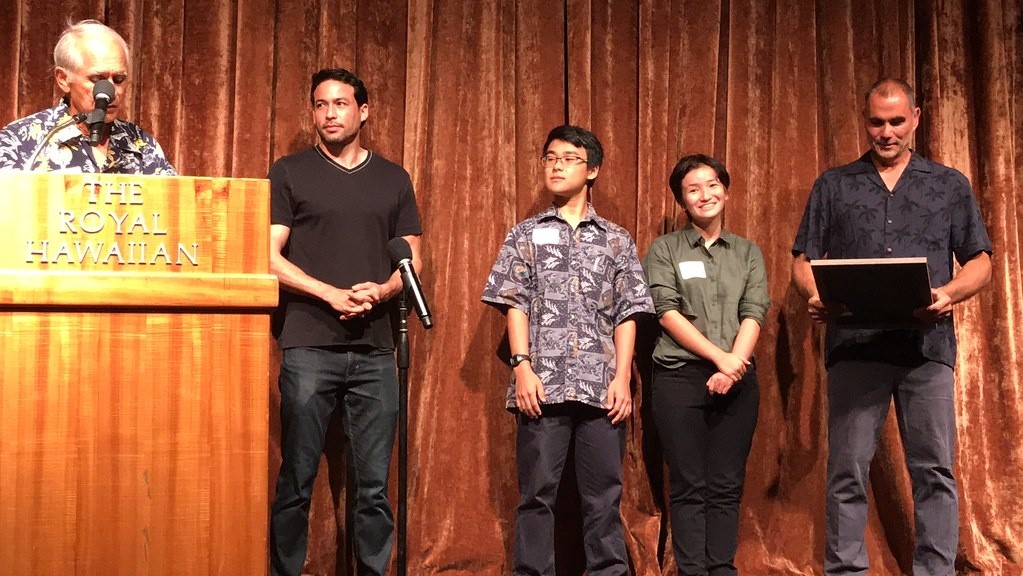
[508,354,532,368]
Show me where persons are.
[789,76,995,576]
[265,68,424,576]
[643,153,772,576]
[1,12,182,175]
[478,124,657,576]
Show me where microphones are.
[386,237,433,330]
[90,79,115,146]
